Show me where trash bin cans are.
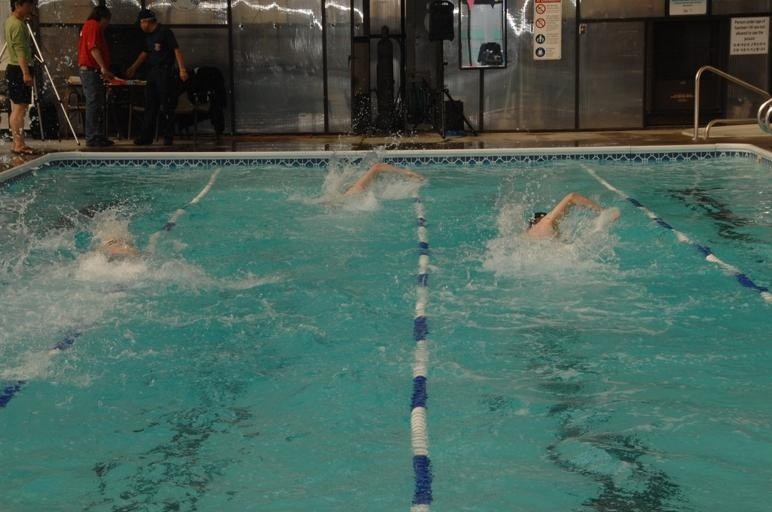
[445,101,465,132]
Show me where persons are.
[529,191,616,236]
[111,243,138,256]
[127,9,189,145]
[345,164,423,193]
[5,0,39,156]
[78,6,114,147]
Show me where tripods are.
[412,41,478,138]
[0,21,82,146]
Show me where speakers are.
[477,43,503,66]
[441,100,466,138]
[424,0,455,42]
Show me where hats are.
[134,9,154,25]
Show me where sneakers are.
[87,135,113,146]
[135,139,153,145]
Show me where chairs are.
[126,64,223,151]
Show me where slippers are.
[11,146,40,155]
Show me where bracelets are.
[180,69,187,72]
